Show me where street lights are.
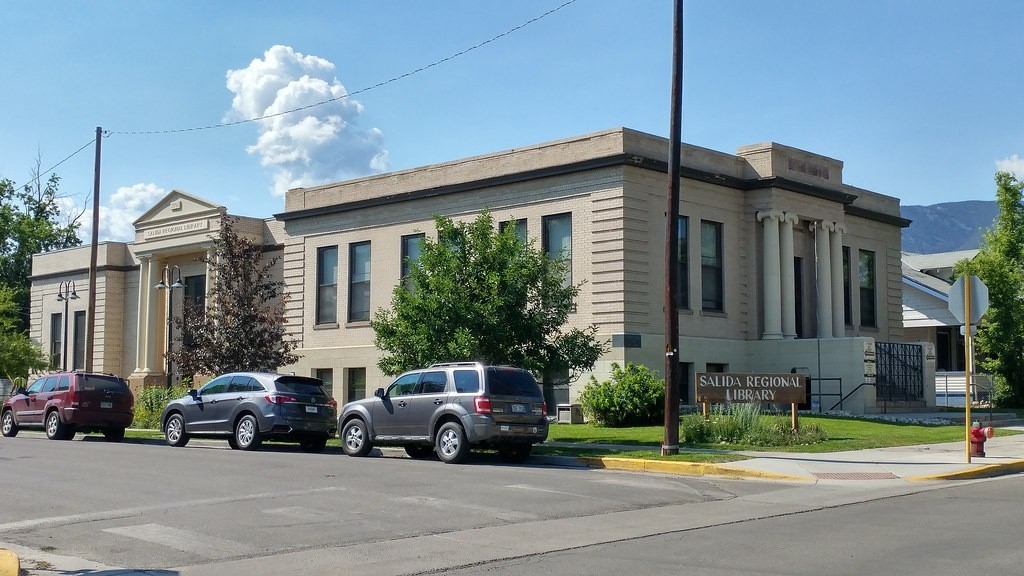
[56,279,80,373]
[154,265,188,399]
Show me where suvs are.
[2,370,136,439]
[161,372,337,452]
[339,362,551,463]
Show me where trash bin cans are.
[556,403,584,425]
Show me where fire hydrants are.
[970,420,995,458]
